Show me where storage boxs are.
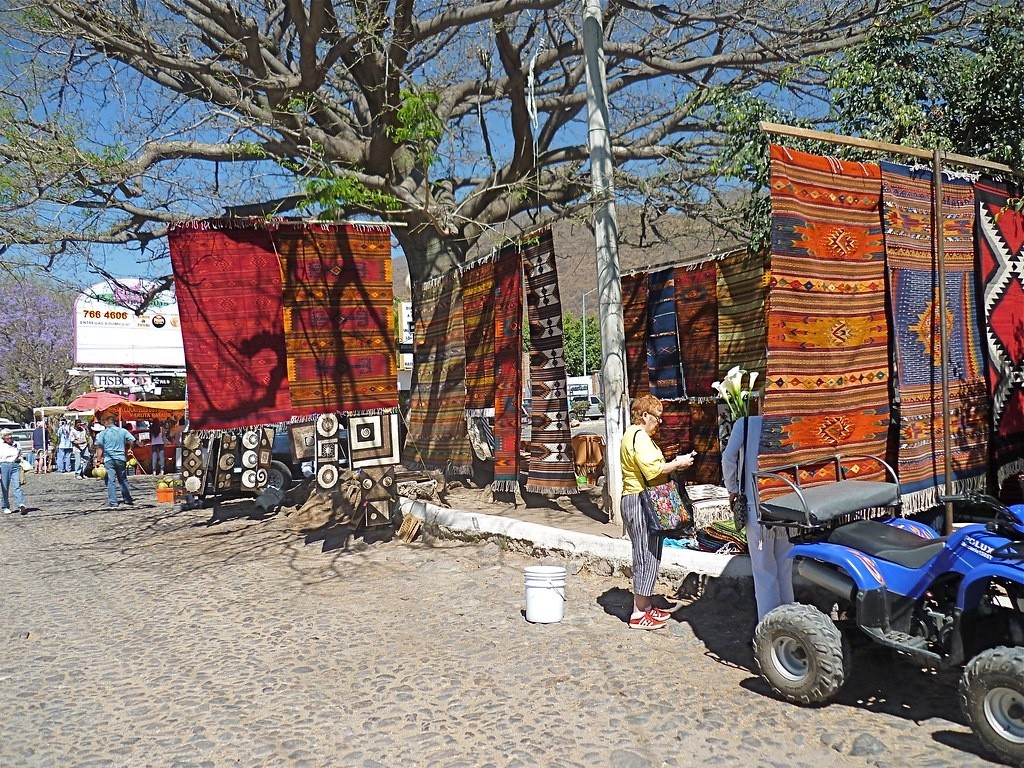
[156,488,182,503]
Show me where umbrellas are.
[65,392,129,412]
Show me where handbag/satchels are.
[633,429,693,537]
[19,460,33,470]
[732,416,748,533]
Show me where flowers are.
[710,364,758,419]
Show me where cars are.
[1,428,54,470]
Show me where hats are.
[91,423,105,431]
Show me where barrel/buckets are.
[524,565,567,623]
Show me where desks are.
[126,445,176,474]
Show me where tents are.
[130,400,185,423]
[33,406,67,475]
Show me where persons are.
[0,429,27,514]
[168,418,185,446]
[149,417,165,477]
[54,419,94,480]
[720,384,794,647]
[126,422,149,448]
[30,420,50,474]
[620,395,695,630]
[95,415,136,507]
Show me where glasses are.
[646,410,662,423]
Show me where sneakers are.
[629,610,667,629]
[649,606,671,621]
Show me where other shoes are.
[46,471,50,473]
[34,469,38,473]
[18,504,27,515]
[107,502,119,507]
[83,475,87,479]
[38,470,44,474]
[3,508,11,513]
[76,475,82,480]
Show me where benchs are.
[752,453,902,530]
